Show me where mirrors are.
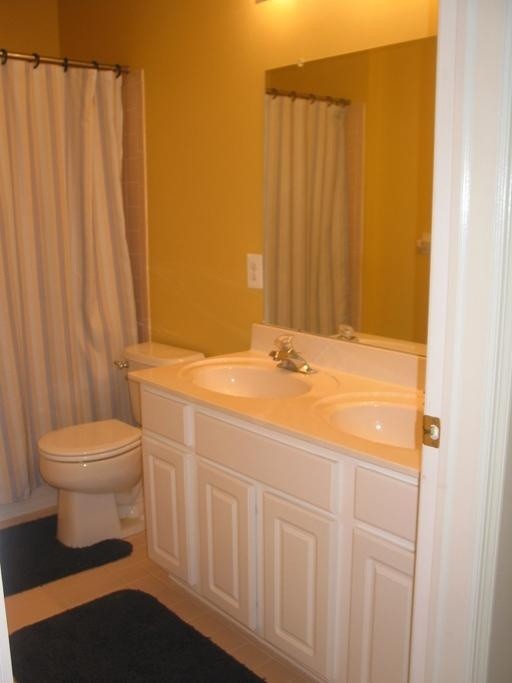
[266,36,436,356]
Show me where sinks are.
[313,393,423,450]
[178,358,309,399]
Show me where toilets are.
[38,342,204,547]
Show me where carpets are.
[0,514,136,597]
[7,585,265,682]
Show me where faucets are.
[269,334,315,375]
[336,325,359,343]
